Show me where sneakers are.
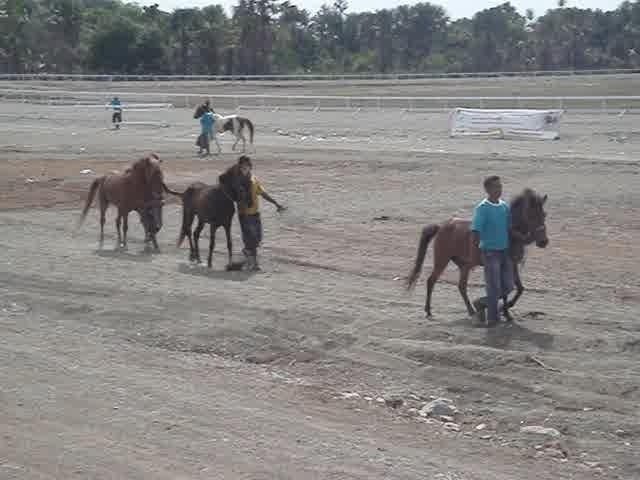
[473,299,498,328]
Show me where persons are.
[235,155,289,274]
[195,104,211,157]
[470,174,516,323]
[105,96,123,129]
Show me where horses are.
[176,166,254,268]
[193,101,254,154]
[78,156,163,249]
[404,187,549,321]
